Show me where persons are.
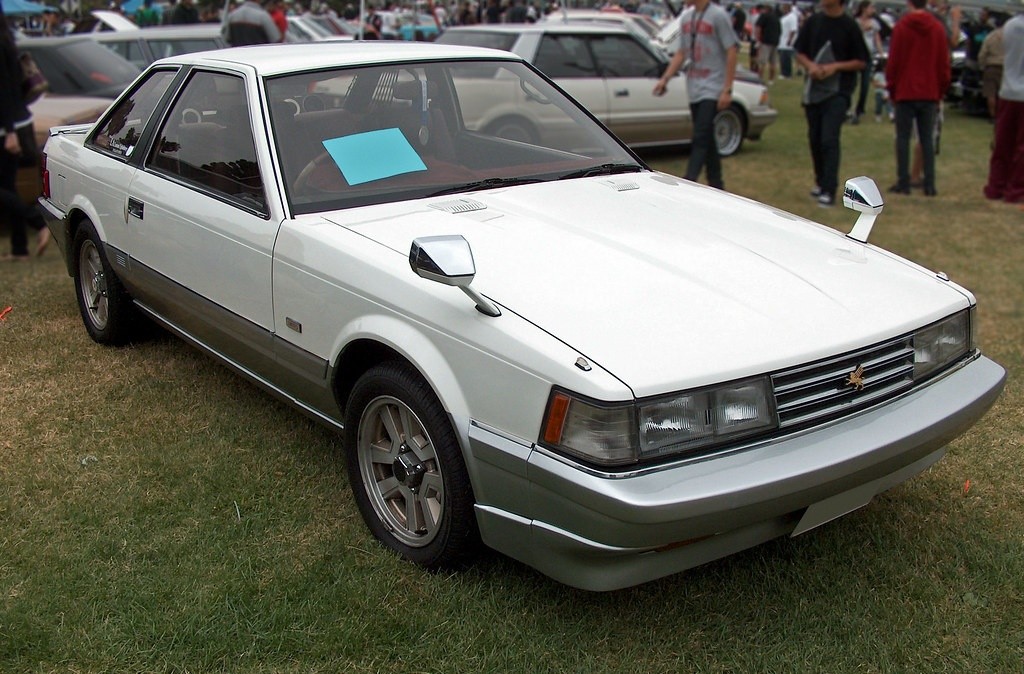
[0,5,53,265]
[792,0,871,209]
[884,0,953,197]
[1,0,1023,125]
[653,0,741,191]
[985,14,1024,203]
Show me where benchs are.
[172,105,364,171]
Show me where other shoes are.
[795,70,803,76]
[1003,193,1024,203]
[922,186,936,197]
[767,80,772,85]
[874,115,882,122]
[817,193,832,205]
[887,183,911,195]
[888,112,895,122]
[785,76,792,81]
[988,116,996,124]
[845,111,852,119]
[0,254,31,261]
[36,226,52,258]
[848,116,860,124]
[778,74,786,80]
[983,185,1003,200]
[911,178,924,186]
[812,186,822,196]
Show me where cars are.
[538,2,680,55]
[41,37,1008,592]
[706,1,1018,125]
[1,2,343,230]
[309,20,775,158]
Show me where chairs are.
[268,100,317,171]
[382,78,460,168]
